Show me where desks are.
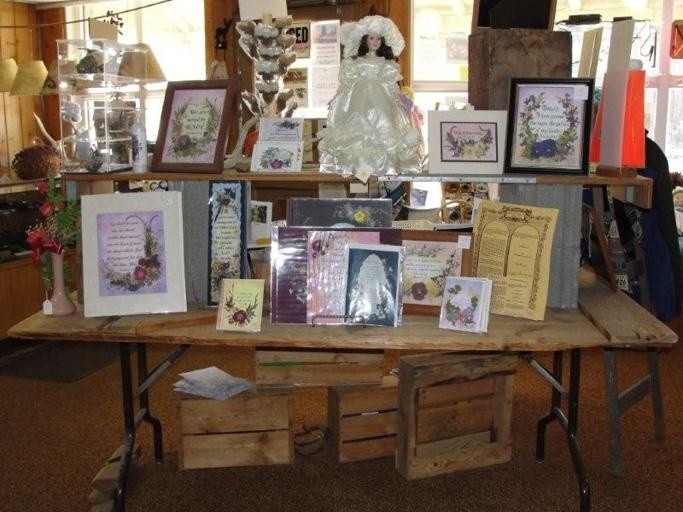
[6,260,680,512]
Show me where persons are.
[317,14,427,180]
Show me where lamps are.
[0,0,177,101]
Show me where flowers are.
[20,161,85,296]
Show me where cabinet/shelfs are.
[53,37,150,176]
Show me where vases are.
[40,249,77,318]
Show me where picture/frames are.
[143,74,240,176]
[501,74,597,179]
[424,108,509,180]
[75,186,188,319]
